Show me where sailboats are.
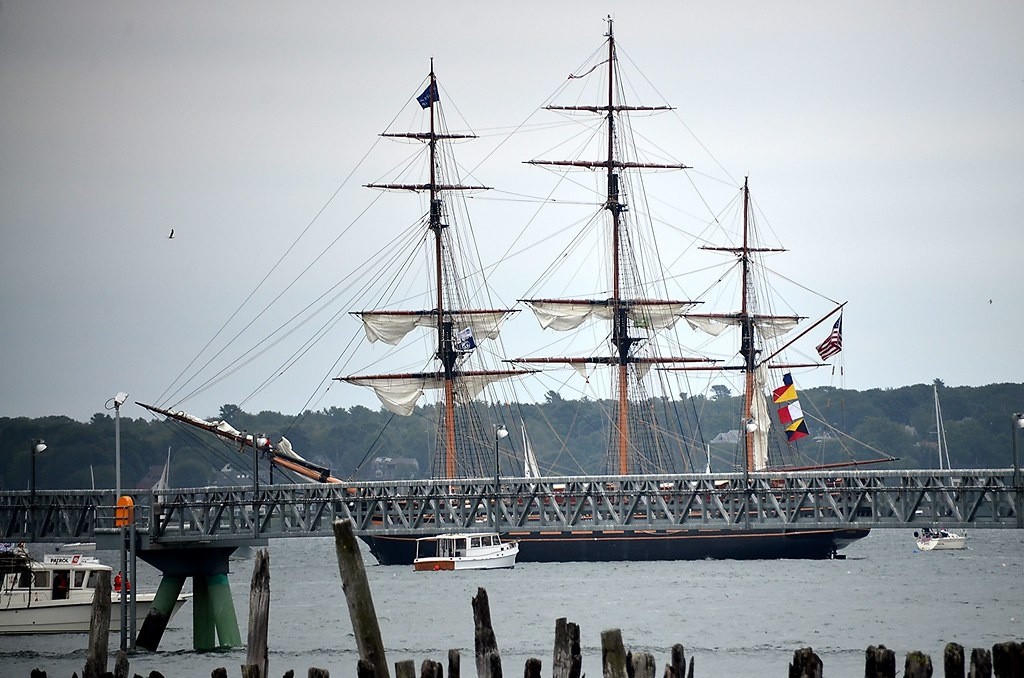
[133,11,906,560]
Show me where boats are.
[414,532,520,571]
[916,530,967,551]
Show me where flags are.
[772,383,799,403]
[416,80,439,110]
[777,400,804,423]
[784,418,810,441]
[815,312,842,361]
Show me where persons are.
[114,571,131,592]
[52,570,68,600]
[260,436,270,461]
[238,430,248,452]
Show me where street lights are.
[1011,410,1024,529]
[495,421,508,523]
[111,391,130,503]
[28,437,49,544]
